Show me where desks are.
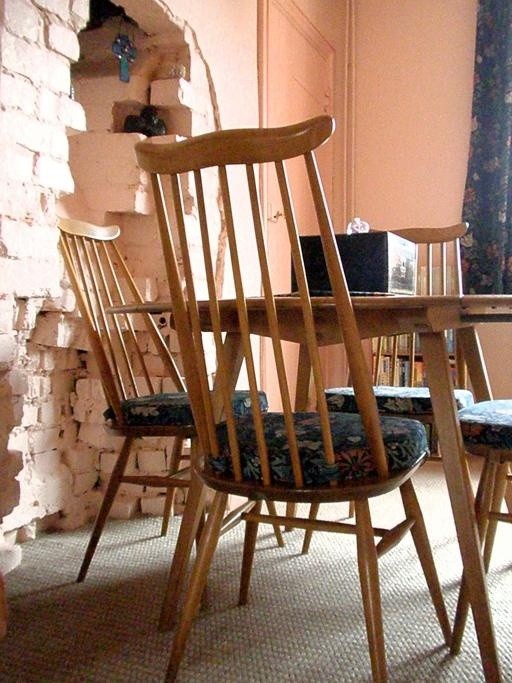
[103,289,512,683]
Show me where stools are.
[445,395,512,659]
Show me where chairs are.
[275,221,469,561]
[52,214,290,617]
[133,113,454,683]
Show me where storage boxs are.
[291,228,421,296]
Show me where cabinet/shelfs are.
[369,348,468,462]
